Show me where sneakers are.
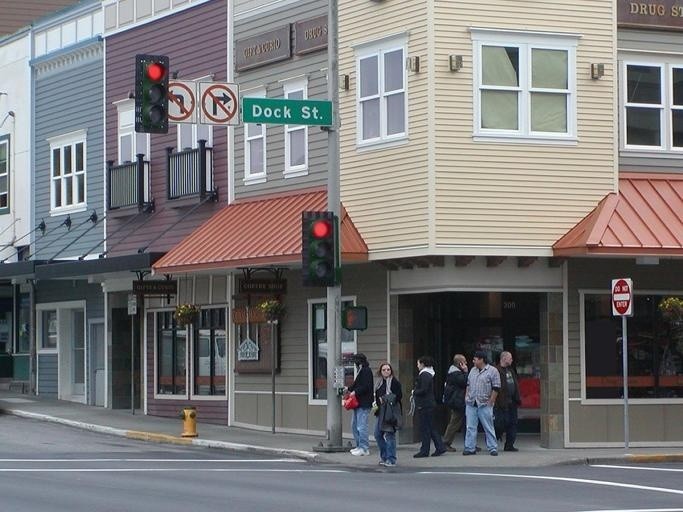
[350,447,370,456]
[431,448,447,456]
[504,446,518,451]
[414,453,428,457]
[445,444,456,452]
[490,451,497,456]
[464,447,481,455]
[379,459,396,467]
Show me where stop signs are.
[611,279,634,318]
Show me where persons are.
[441,354,482,452]
[343,353,374,456]
[485,351,521,451]
[372,362,403,466]
[463,352,502,456]
[411,357,448,457]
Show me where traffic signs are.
[242,97,333,128]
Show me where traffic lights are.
[340,307,368,332]
[300,209,336,288]
[136,55,169,135]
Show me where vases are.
[264,312,288,321]
[662,312,683,326]
[176,314,192,325]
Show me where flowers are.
[173,302,200,319]
[255,297,289,314]
[657,297,683,322]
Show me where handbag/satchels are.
[407,398,415,417]
[344,394,359,411]
[370,406,378,417]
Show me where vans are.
[161,328,226,397]
[312,339,356,391]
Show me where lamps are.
[449,54,463,72]
[338,73,349,89]
[406,55,419,72]
[591,63,604,78]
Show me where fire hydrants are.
[179,406,200,439]
